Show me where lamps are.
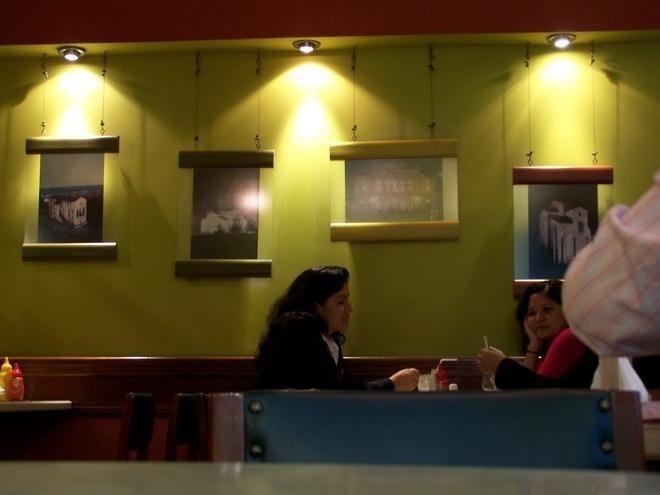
[58,48,86,62]
[293,40,321,53]
[547,34,577,50]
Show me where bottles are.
[0,357,24,402]
[430,364,449,391]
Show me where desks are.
[1,401,72,465]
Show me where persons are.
[562,172,660,360]
[477,281,599,389]
[250,266,420,392]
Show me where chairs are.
[210,388,650,468]
[165,394,207,461]
[116,391,153,461]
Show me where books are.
[642,400,659,460]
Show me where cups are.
[482,370,497,392]
[418,375,431,392]
